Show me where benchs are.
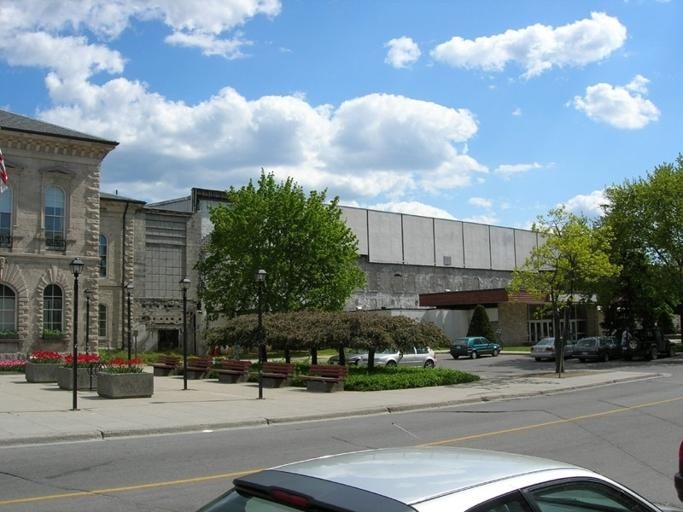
[153,354,348,393]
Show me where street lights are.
[178,277,193,390]
[69,257,85,411]
[83,288,94,356]
[254,265,268,399]
[123,280,135,368]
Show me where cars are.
[328,346,437,369]
[195,441,683,512]
[530,327,676,362]
[450,336,501,359]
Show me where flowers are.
[105,356,144,376]
[28,348,66,365]
[66,350,101,368]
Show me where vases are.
[25,363,63,383]
[57,365,155,399]
